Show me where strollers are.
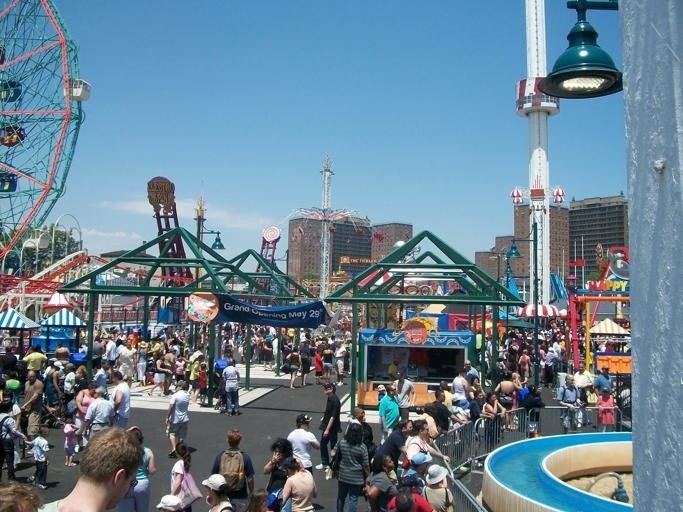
[38,400,65,430]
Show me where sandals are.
[464,458,484,468]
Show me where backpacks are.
[218,449,245,491]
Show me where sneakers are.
[35,482,47,490]
[74,444,81,453]
[315,463,327,470]
[335,381,344,387]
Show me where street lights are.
[494,253,513,319]
[193,195,225,348]
[507,220,540,390]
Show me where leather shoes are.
[227,411,239,416]
[168,450,176,457]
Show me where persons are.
[0,315,631,512]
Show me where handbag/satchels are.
[401,454,411,470]
[324,462,337,481]
[170,471,203,511]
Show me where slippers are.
[65,462,77,467]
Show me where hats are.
[409,452,432,466]
[174,381,189,389]
[296,413,313,424]
[451,393,463,402]
[137,340,149,348]
[156,494,186,512]
[377,384,385,392]
[424,463,449,485]
[201,473,229,492]
